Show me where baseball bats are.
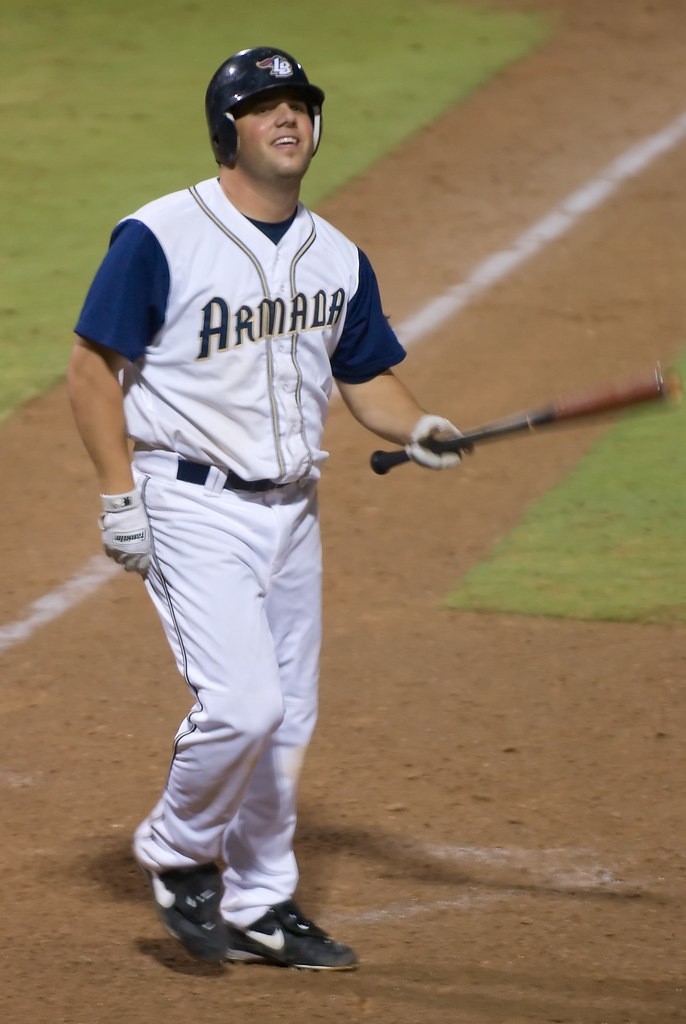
[367,371,670,480]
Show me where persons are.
[64,44,477,972]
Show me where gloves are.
[403,415,475,468]
[98,489,151,575]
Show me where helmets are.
[205,45,324,171]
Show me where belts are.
[177,460,290,491]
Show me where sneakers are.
[227,901,358,969]
[141,864,227,964]
